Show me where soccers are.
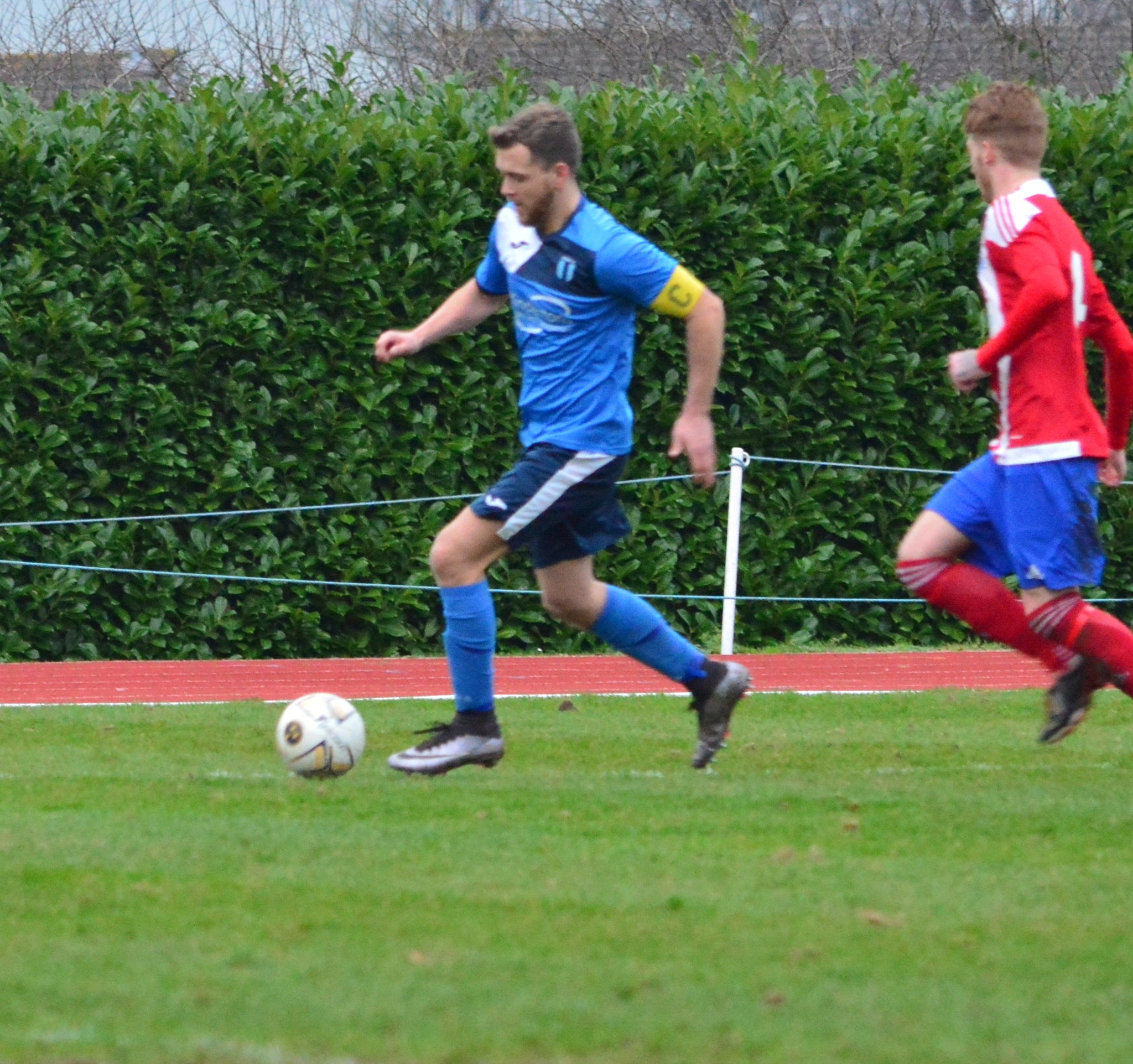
[275,690,367,781]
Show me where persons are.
[370,105,750,774]
[893,86,1133,741]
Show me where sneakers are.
[1034,656,1109,743]
[690,659,754,769]
[391,713,503,773]
